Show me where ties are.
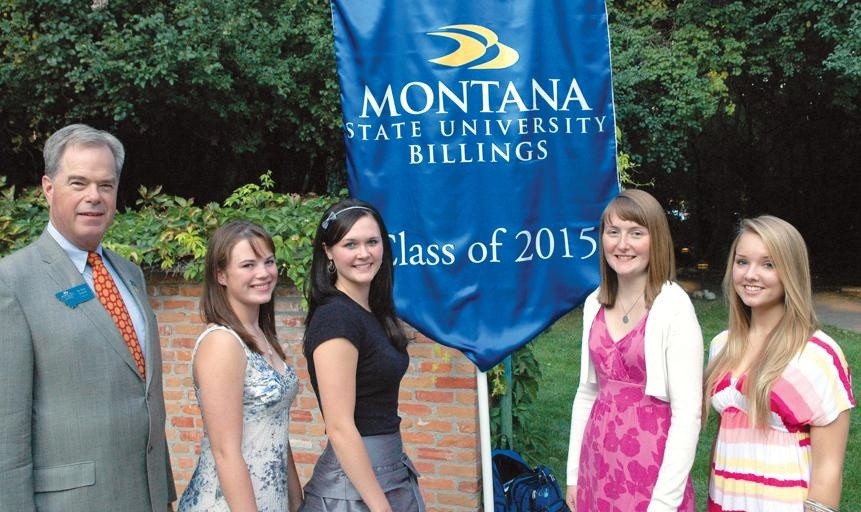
[85,251,146,381]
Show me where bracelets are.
[803,498,838,512]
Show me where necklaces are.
[617,291,644,324]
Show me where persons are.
[176,216,303,511]
[706,215,856,512]
[566,189,704,512]
[1,123,180,512]
[299,198,426,512]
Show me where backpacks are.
[492,434,572,512]
